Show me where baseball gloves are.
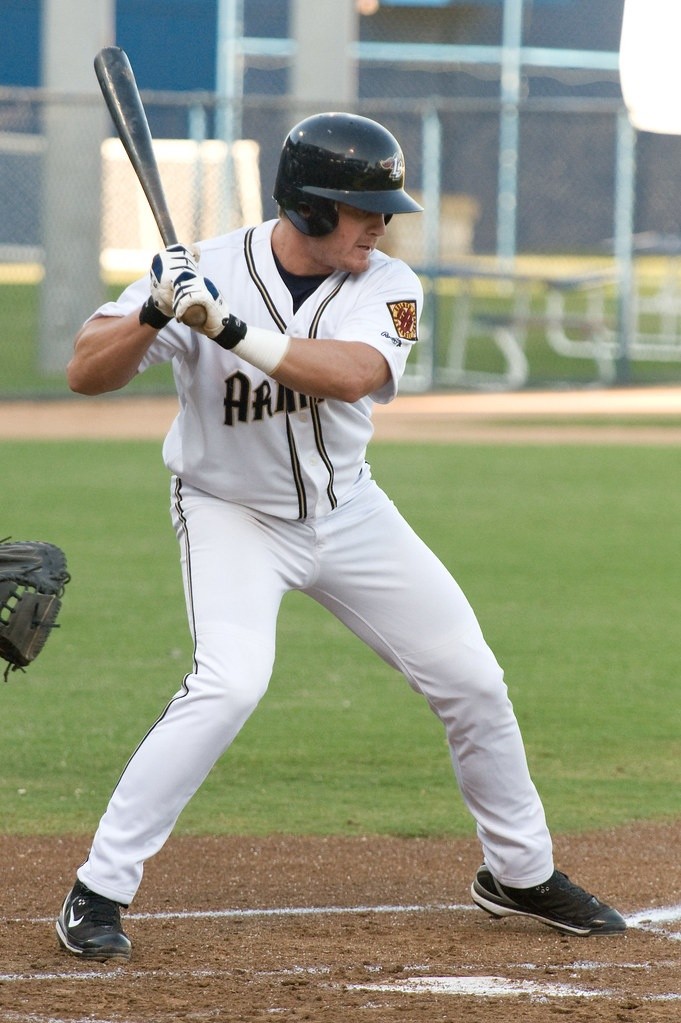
[0,540,70,666]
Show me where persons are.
[53,112,625,955]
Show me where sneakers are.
[54,879,132,960]
[471,861,627,936]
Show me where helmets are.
[273,112,424,238]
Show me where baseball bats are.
[94,45,208,329]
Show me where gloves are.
[138,243,199,329]
[171,270,248,351]
